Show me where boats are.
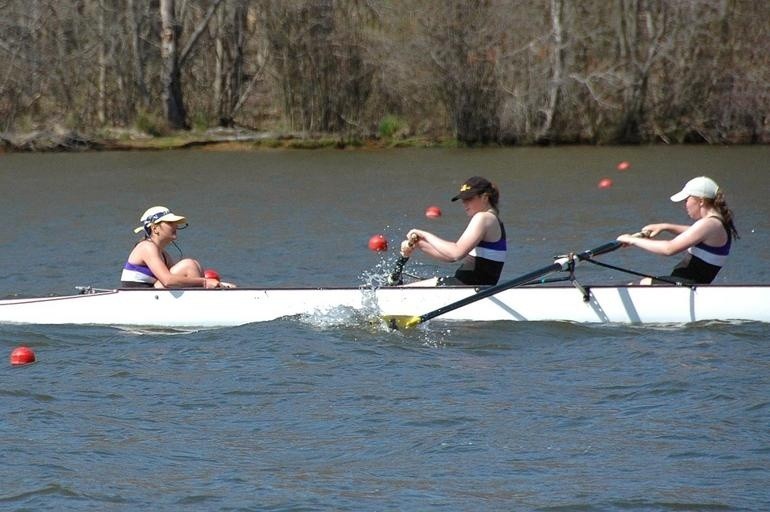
[0,286,768,338]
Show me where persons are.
[613,175,741,285]
[390,176,507,287]
[119,205,238,287]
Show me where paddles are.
[374,232,653,331]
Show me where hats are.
[451,176,495,201]
[669,176,719,202]
[135,206,188,235]
